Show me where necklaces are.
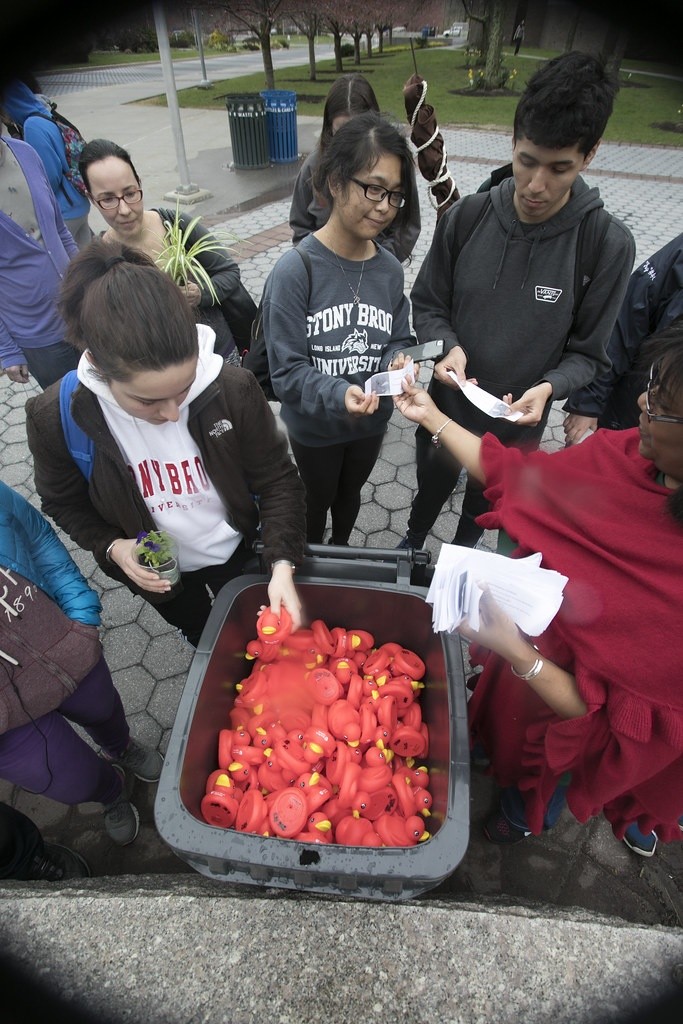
[325,228,365,305]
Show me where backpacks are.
[23,102,89,207]
[239,247,406,404]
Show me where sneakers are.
[484,806,550,845]
[22,841,91,882]
[468,733,492,765]
[101,735,165,783]
[99,763,140,848]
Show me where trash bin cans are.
[260,89,299,164]
[221,90,269,169]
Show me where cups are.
[131,531,180,585]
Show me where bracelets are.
[430,418,453,449]
[510,644,544,681]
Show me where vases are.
[132,531,181,587]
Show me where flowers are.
[135,530,175,565]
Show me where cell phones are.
[391,339,445,366]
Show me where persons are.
[289,73,421,263]
[0,479,165,882]
[25,240,306,651]
[513,17,525,56]
[394,49,683,858]
[263,114,421,547]
[0,79,258,390]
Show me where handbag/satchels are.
[160,206,258,355]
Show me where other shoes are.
[678,815,683,832]
[328,537,355,559]
[381,536,425,568]
[622,820,659,858]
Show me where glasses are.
[645,361,683,424]
[347,175,406,209]
